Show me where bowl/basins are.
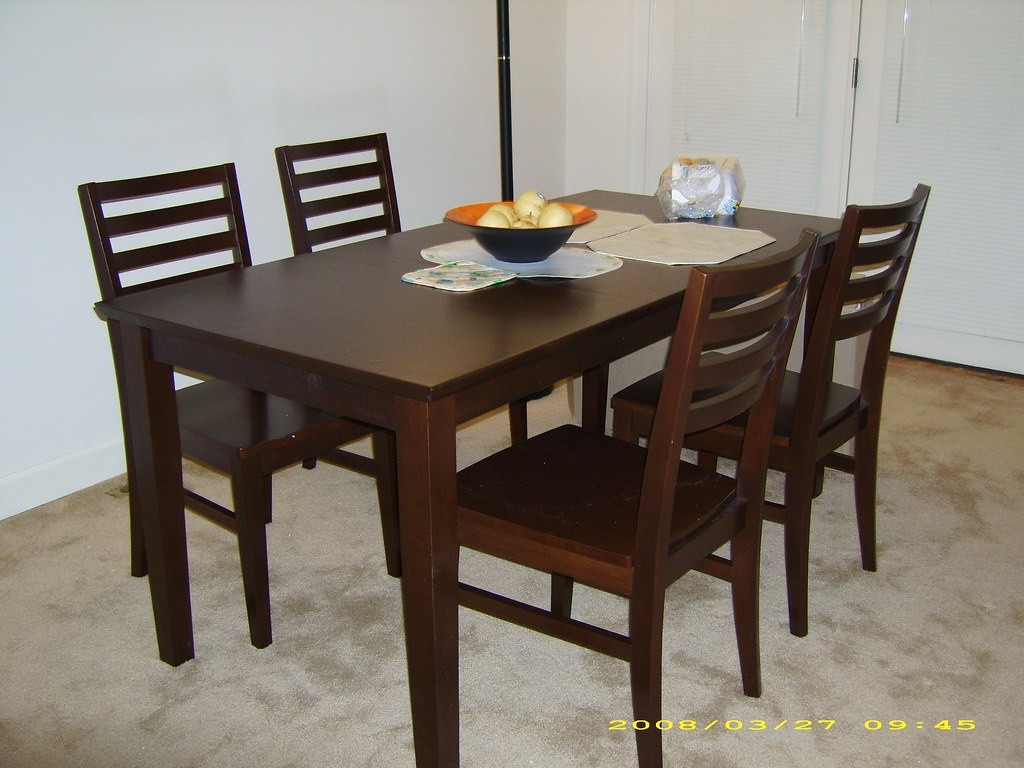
[445,202,599,264]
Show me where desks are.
[96,185,826,768]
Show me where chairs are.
[273,132,527,573]
[79,160,400,649]
[609,183,932,639]
[447,229,821,768]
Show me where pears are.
[476,191,573,229]
